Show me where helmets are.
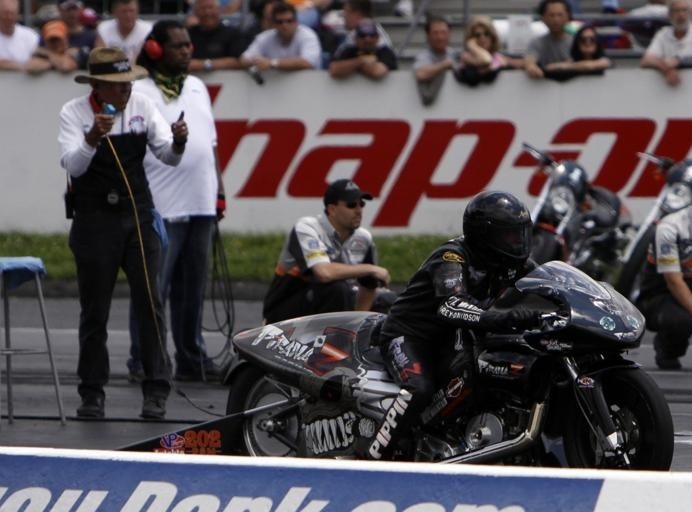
[463,191,534,265]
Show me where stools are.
[0,254,66,424]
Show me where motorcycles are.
[518,142,632,278]
[614,149,692,318]
[219,259,675,470]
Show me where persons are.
[521,0,612,77]
[261,180,390,326]
[634,205,692,370]
[596,0,671,49]
[0,0,152,73]
[57,47,189,419]
[641,0,691,86]
[125,19,226,385]
[362,190,615,460]
[413,14,523,80]
[186,0,412,79]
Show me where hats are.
[354,17,377,35]
[43,21,68,41]
[325,179,372,201]
[75,47,150,83]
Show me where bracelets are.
[172,136,188,145]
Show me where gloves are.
[477,309,541,334]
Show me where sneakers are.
[127,359,145,383]
[652,334,683,371]
[143,385,168,418]
[77,389,104,416]
[175,351,222,381]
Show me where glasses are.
[347,202,365,208]
